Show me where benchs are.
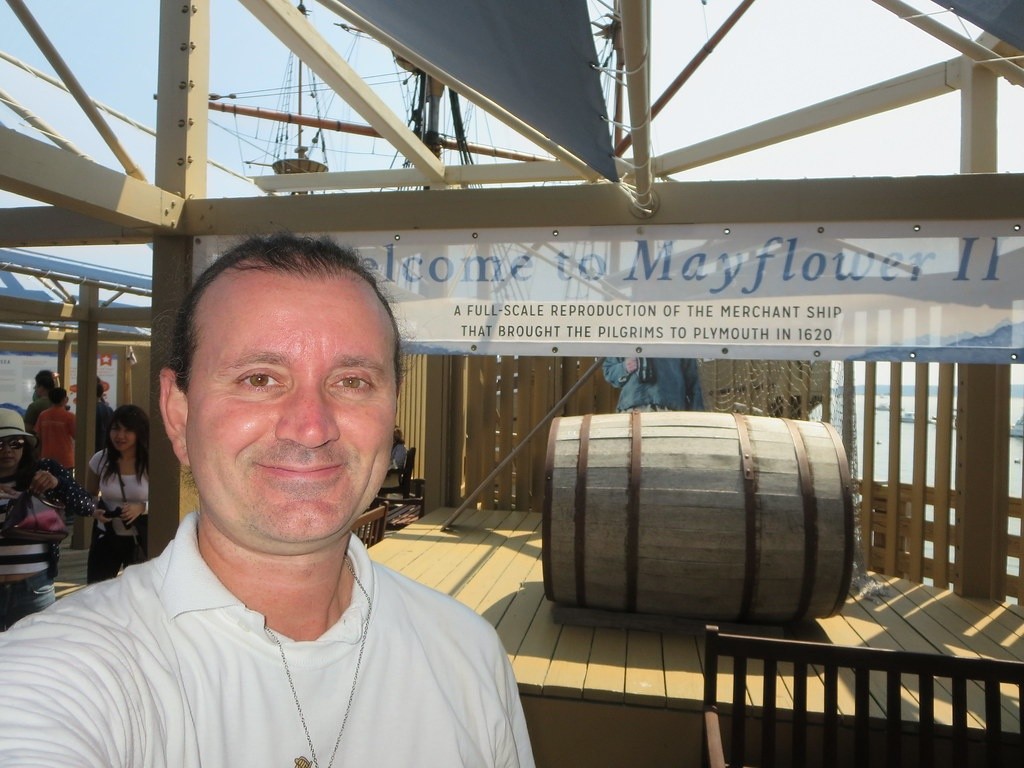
[699,625,1024,768]
[370,478,426,531]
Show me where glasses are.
[0,437,25,450]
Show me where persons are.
[0,369,150,632]
[603,358,704,411]
[389,429,407,468]
[0,231,536,768]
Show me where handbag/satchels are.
[111,516,139,538]
[0,489,69,542]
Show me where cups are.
[630,358,657,385]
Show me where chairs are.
[377,447,416,499]
[350,501,389,550]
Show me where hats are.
[0,407,37,449]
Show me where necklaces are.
[266,557,371,767]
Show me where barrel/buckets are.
[542,411,855,624]
